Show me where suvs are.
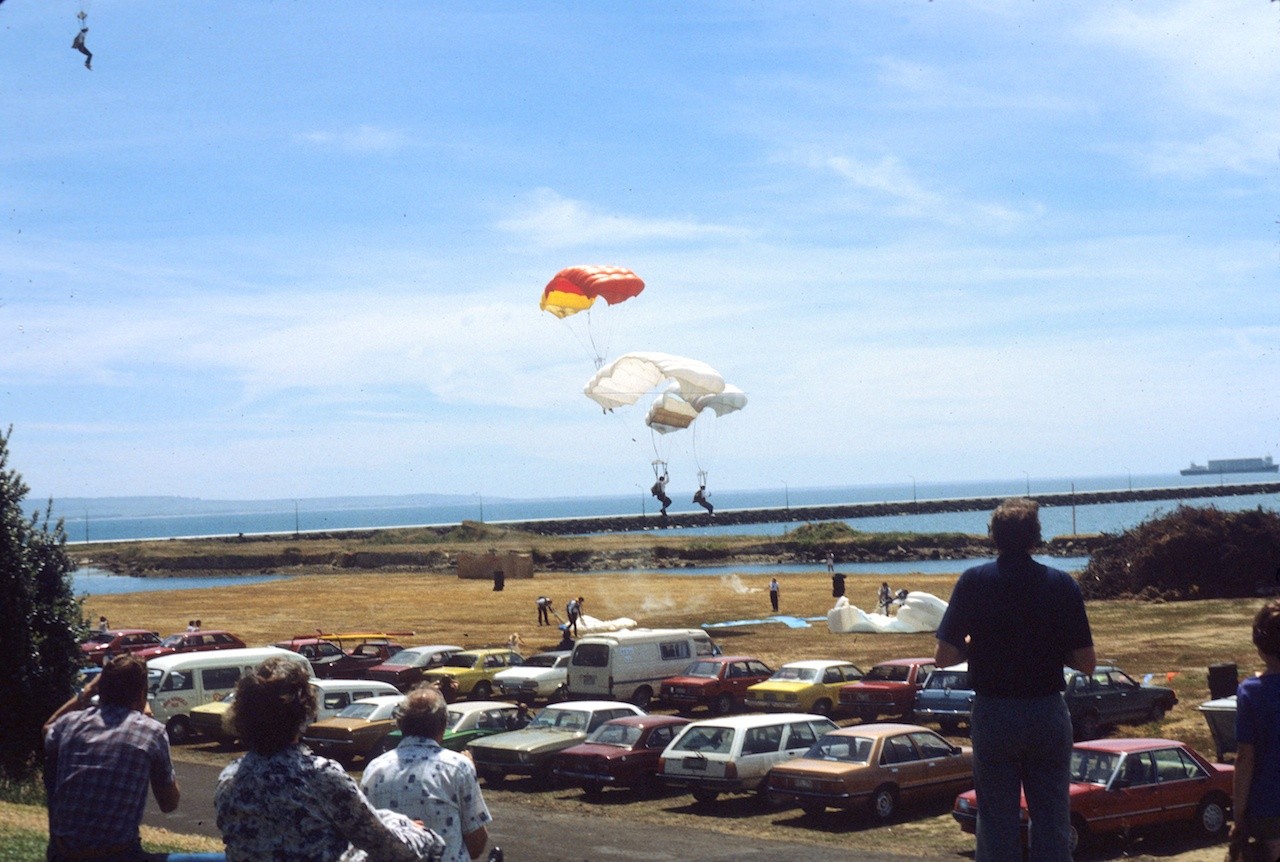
[134,630,246,669]
[78,629,167,667]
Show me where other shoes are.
[487,846,504,862]
[660,509,667,516]
[709,513,715,518]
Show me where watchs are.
[76,688,89,704]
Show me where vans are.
[148,644,316,745]
[563,627,730,707]
[309,678,404,719]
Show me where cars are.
[951,737,1234,843]
[187,687,247,747]
[744,660,865,718]
[1059,667,1180,728]
[917,663,973,729]
[368,645,467,696]
[493,651,578,706]
[327,640,406,677]
[661,656,796,716]
[387,703,554,755]
[654,713,900,804]
[306,696,410,766]
[837,658,938,724]
[463,701,650,784]
[553,715,712,795]
[422,648,531,703]
[768,723,975,821]
[278,633,355,673]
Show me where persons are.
[42,654,180,862]
[768,578,780,612]
[657,472,673,516]
[825,550,836,572]
[935,499,1097,862]
[1229,604,1280,862]
[77,27,92,70]
[187,620,202,632]
[694,486,716,518]
[536,596,553,626]
[99,616,110,630]
[362,688,492,862]
[566,597,584,639]
[556,630,575,651]
[213,657,446,862]
[877,582,893,616]
